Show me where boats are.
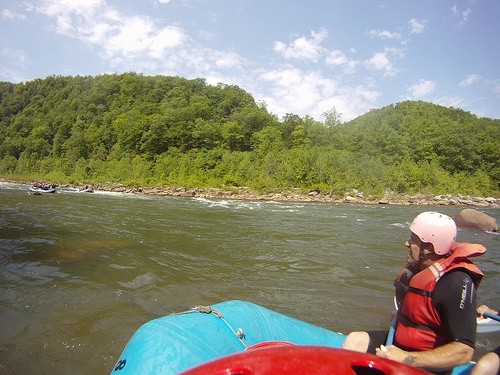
[79,188,94,193]
[109,300,478,375]
[29,186,57,193]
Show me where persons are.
[341,212,485,375]
[466,305,500,375]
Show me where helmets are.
[409,211,457,269]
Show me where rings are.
[479,308,482,311]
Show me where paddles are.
[483,312,500,322]
[385,314,397,346]
